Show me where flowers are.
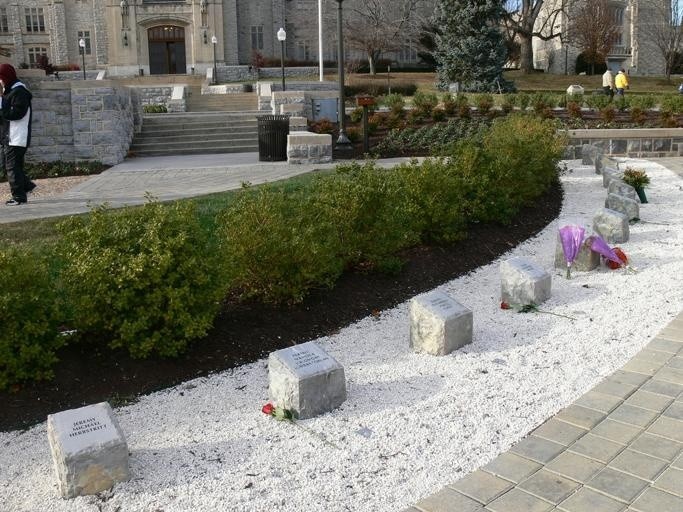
[620,165,652,206]
[259,402,343,451]
[498,299,578,322]
[558,223,637,282]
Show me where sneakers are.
[5,196,27,206]
[25,182,39,196]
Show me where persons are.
[603,67,615,97]
[615,68,629,95]
[1,63,36,205]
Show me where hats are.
[607,67,612,70]
[0,63,17,84]
[619,68,625,72]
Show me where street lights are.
[277,28,287,90]
[79,38,86,80]
[212,35,218,82]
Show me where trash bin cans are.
[256,115,290,161]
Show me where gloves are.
[610,86,613,90]
[626,86,629,90]
